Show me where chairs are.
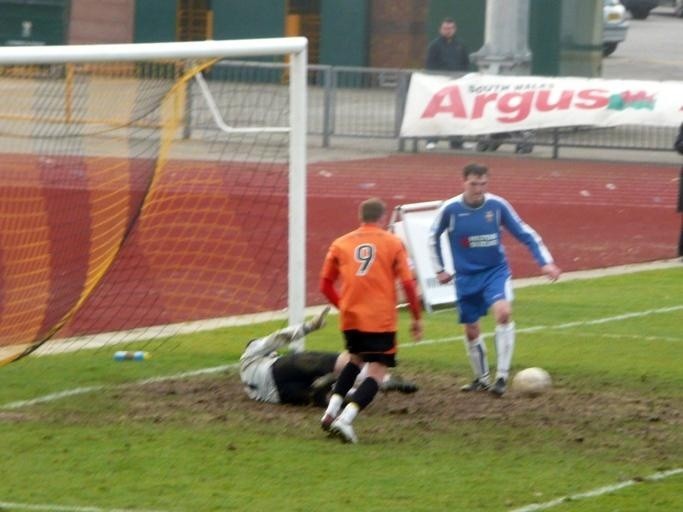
[381,375,419,396]
[320,411,357,445]
[459,374,509,398]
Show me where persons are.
[422,18,476,152]
[238,304,419,407]
[319,196,424,445]
[426,161,563,399]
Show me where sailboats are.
[512,367,551,399]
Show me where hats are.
[603,0,631,56]
